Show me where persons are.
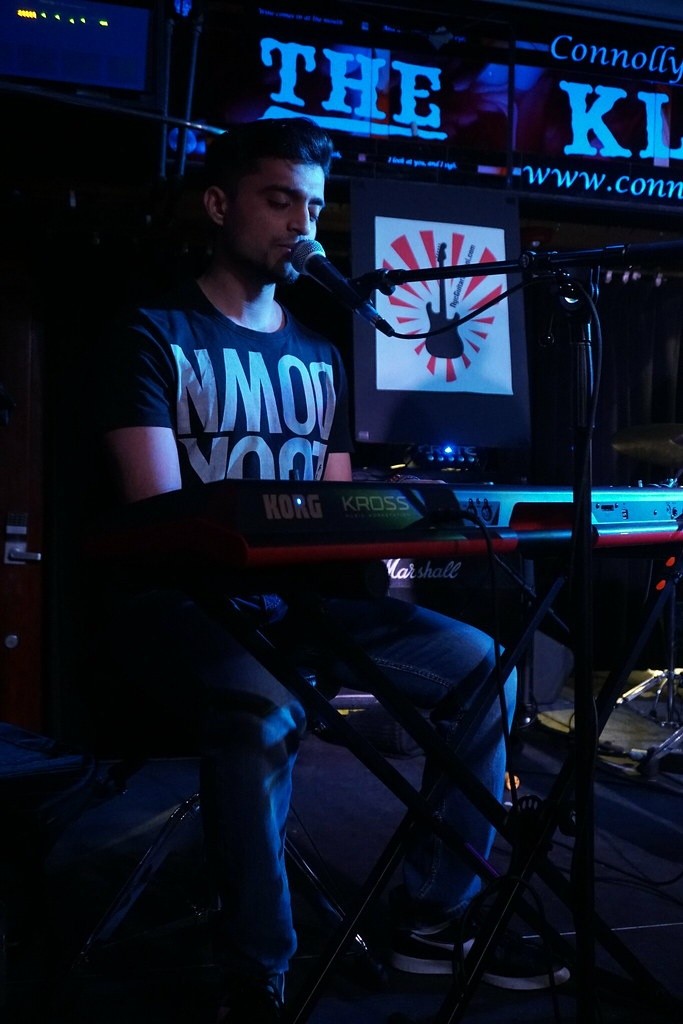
[103,116,576,1024]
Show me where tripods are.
[612,551,683,730]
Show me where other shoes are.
[387,912,570,991]
[219,963,293,1024]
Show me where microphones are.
[289,238,394,338]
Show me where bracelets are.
[388,473,421,483]
